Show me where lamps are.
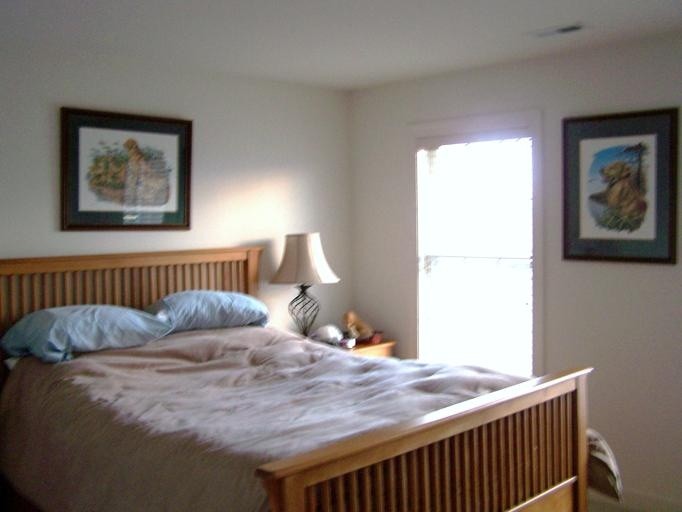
[269,232,341,336]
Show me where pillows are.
[0,304,173,363]
[145,290,269,334]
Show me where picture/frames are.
[58,106,191,233]
[561,106,676,265]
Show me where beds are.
[0,245,624,512]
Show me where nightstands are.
[338,332,397,358]
